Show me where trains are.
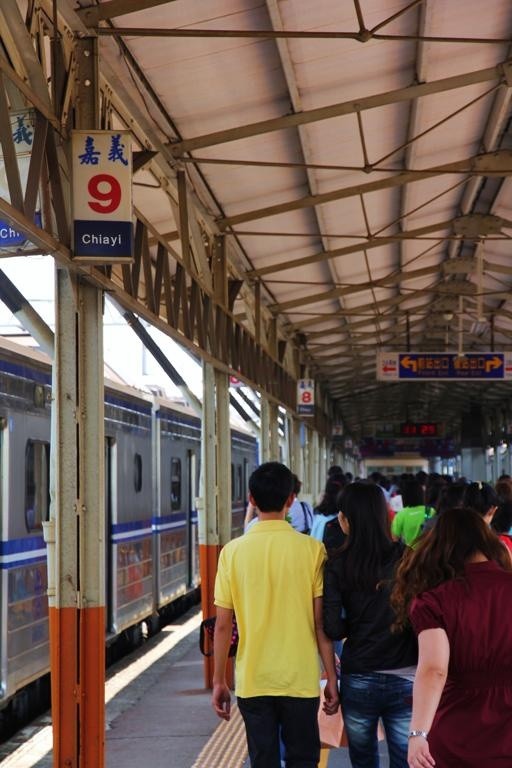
[0,329,291,705]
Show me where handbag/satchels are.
[318,689,385,748]
[199,614,239,657]
